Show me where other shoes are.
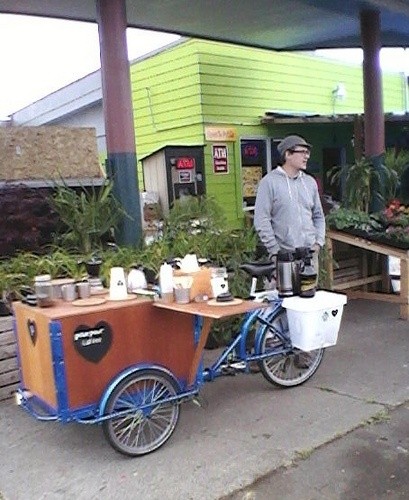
[293,354,309,369]
[278,356,291,373]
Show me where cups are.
[174,287,190,304]
[75,283,91,299]
[61,283,76,300]
[89,278,104,291]
[109,267,129,298]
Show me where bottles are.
[33,275,53,308]
[159,260,175,303]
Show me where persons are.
[254,136,326,373]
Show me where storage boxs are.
[282,290,347,353]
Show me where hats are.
[277,135,313,157]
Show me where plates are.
[207,298,243,306]
[73,298,106,306]
[88,289,109,295]
[108,295,137,302]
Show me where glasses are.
[290,150,311,156]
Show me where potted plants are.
[325,205,409,250]
[0,166,265,356]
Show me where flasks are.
[270,248,295,298]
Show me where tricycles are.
[9,244,326,459]
